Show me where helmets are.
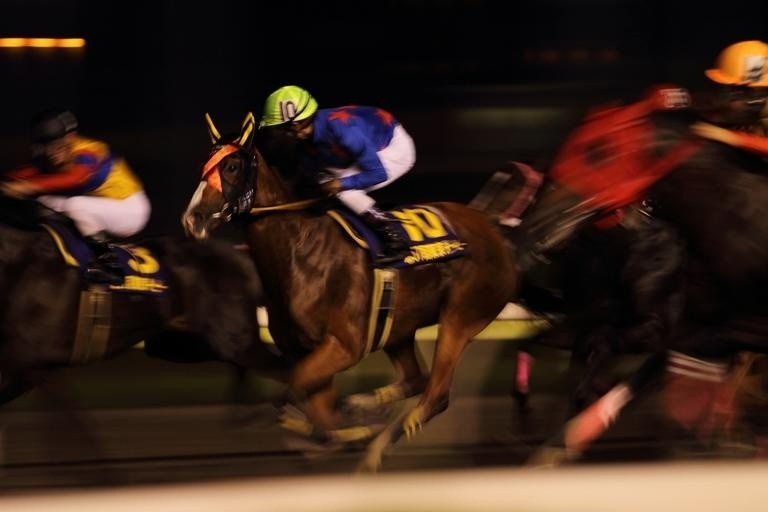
[27,104,79,148]
[258,84,319,131]
[702,39,768,88]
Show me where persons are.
[691,37,767,179]
[259,84,420,268]
[0,100,154,286]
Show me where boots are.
[360,200,412,264]
[78,230,125,285]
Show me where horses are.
[0,178,292,411]
[181,109,581,476]
[464,86,768,475]
